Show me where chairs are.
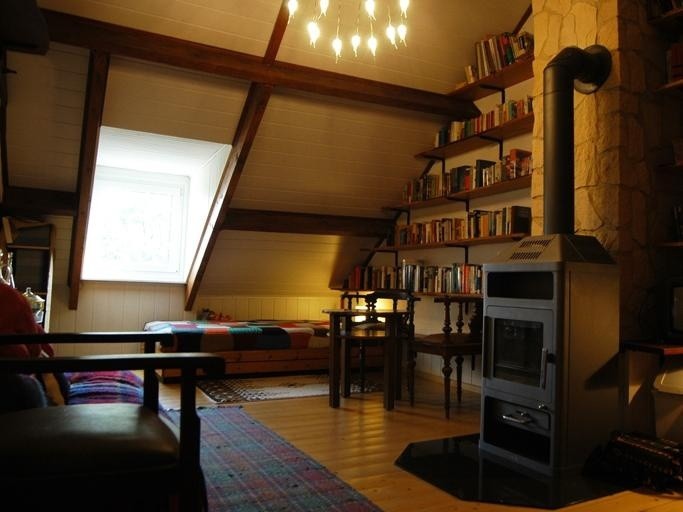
[340,289,482,419]
[0,332,226,511]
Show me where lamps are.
[286,0,412,64]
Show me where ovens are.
[482,261,623,477]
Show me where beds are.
[145,318,388,384]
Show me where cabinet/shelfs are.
[331,56,533,303]
[1,218,57,332]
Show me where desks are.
[321,309,414,411]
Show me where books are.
[455,32,534,90]
[402,149,532,204]
[394,205,531,247]
[348,258,483,294]
[434,95,532,148]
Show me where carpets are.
[170,405,382,512]
[196,373,381,405]
